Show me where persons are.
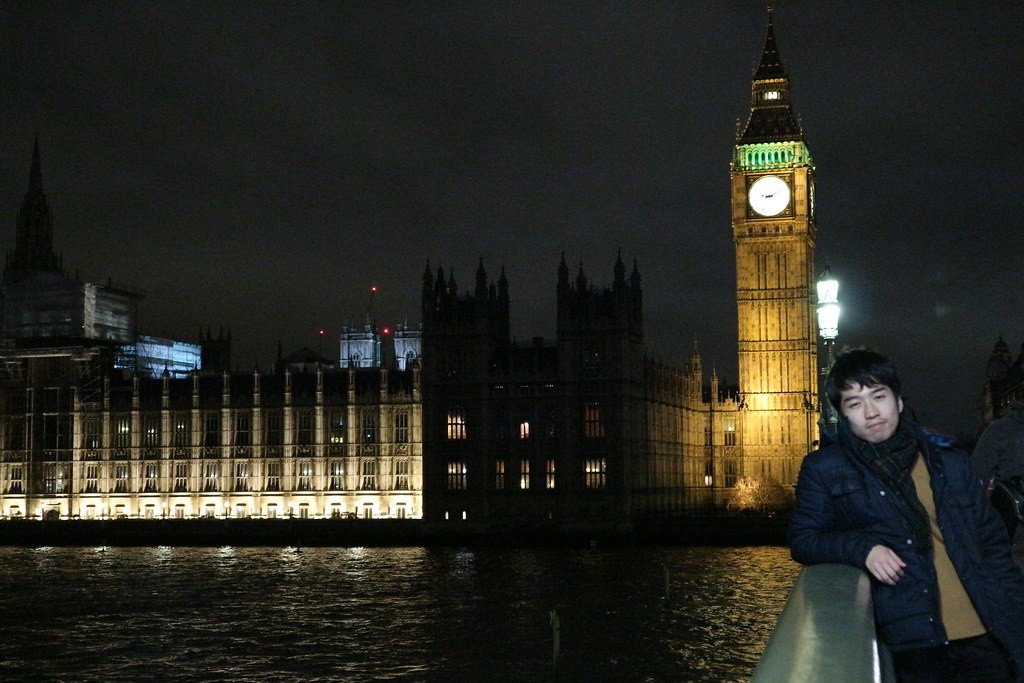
[789,349,1024,683]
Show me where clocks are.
[747,175,793,218]
[809,182,816,219]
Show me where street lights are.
[815,264,842,436]
